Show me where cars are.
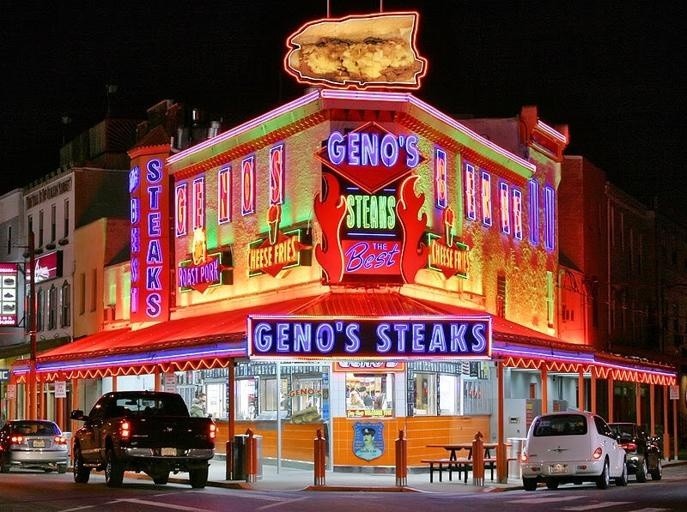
[0,419,69,474]
[520,410,628,491]
[607,422,663,483]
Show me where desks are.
[426,442,512,484]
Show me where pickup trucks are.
[70,390,217,488]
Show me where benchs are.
[421,456,518,483]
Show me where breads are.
[299,37,421,83]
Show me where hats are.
[361,428,375,436]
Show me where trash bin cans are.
[506,438,528,483]
[232,434,263,481]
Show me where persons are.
[355,427,383,460]
[346,385,385,410]
[189,393,210,418]
